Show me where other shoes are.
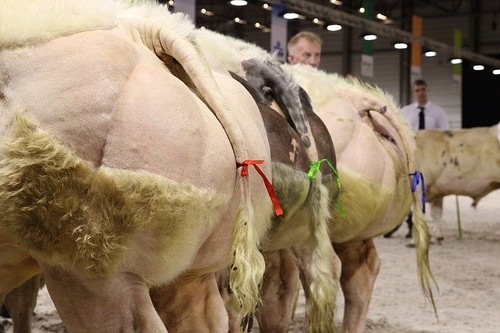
[384,225,400,238]
[406,231,412,238]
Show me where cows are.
[0,1,440,333]
[385,123,499,247]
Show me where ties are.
[417,106,425,129]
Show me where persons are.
[401,79,450,238]
[287,32,323,70]
